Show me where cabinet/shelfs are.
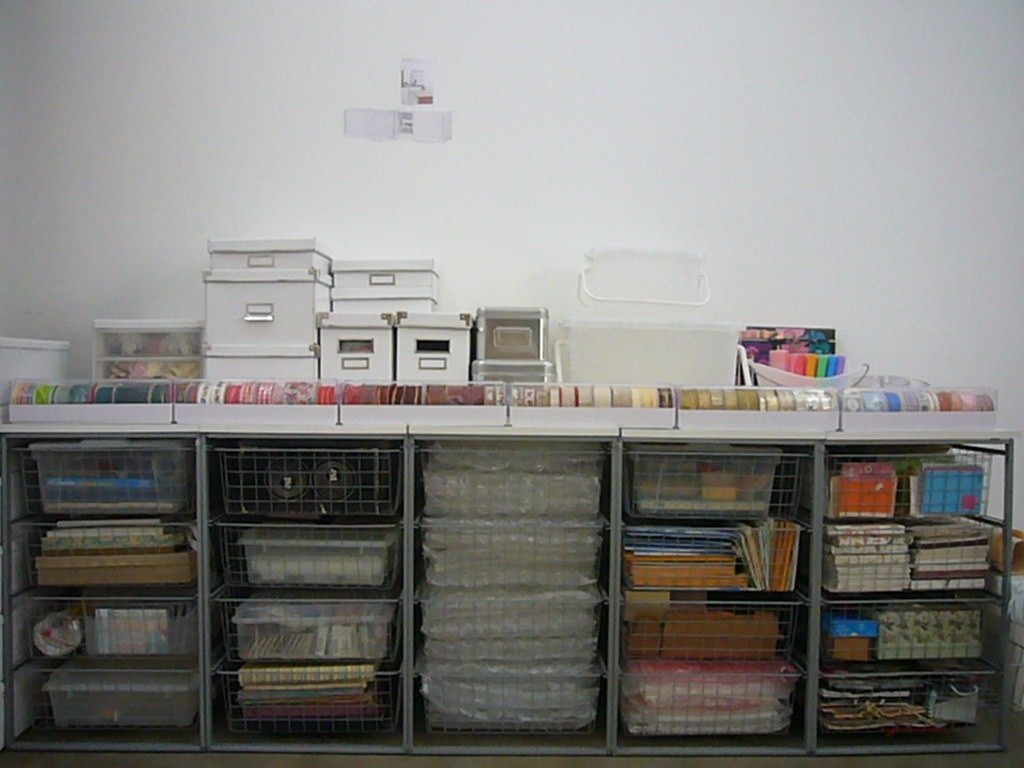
[2,425,1018,763]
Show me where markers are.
[789,351,847,377]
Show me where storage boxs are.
[239,527,401,589]
[43,661,200,729]
[627,444,783,520]
[76,601,200,656]
[624,613,776,663]
[85,238,737,392]
[32,441,186,517]
[820,615,878,660]
[242,600,393,665]
[868,610,982,658]
[423,446,601,731]
[0,335,70,424]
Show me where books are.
[829,463,897,517]
[623,516,801,591]
[41,527,186,556]
[822,523,911,593]
[244,613,386,659]
[237,664,377,718]
[910,465,983,517]
[816,678,923,730]
[619,661,792,736]
[911,525,990,591]
